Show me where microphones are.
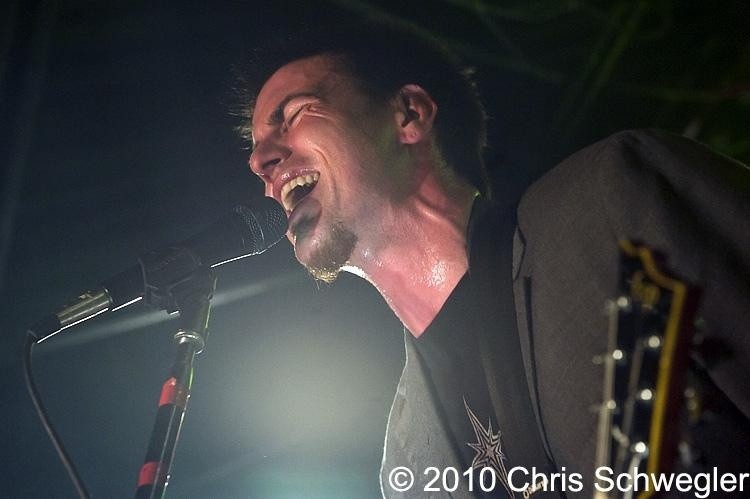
[26,196,289,344]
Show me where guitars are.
[596,242,711,498]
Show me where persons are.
[227,0,750,499]
